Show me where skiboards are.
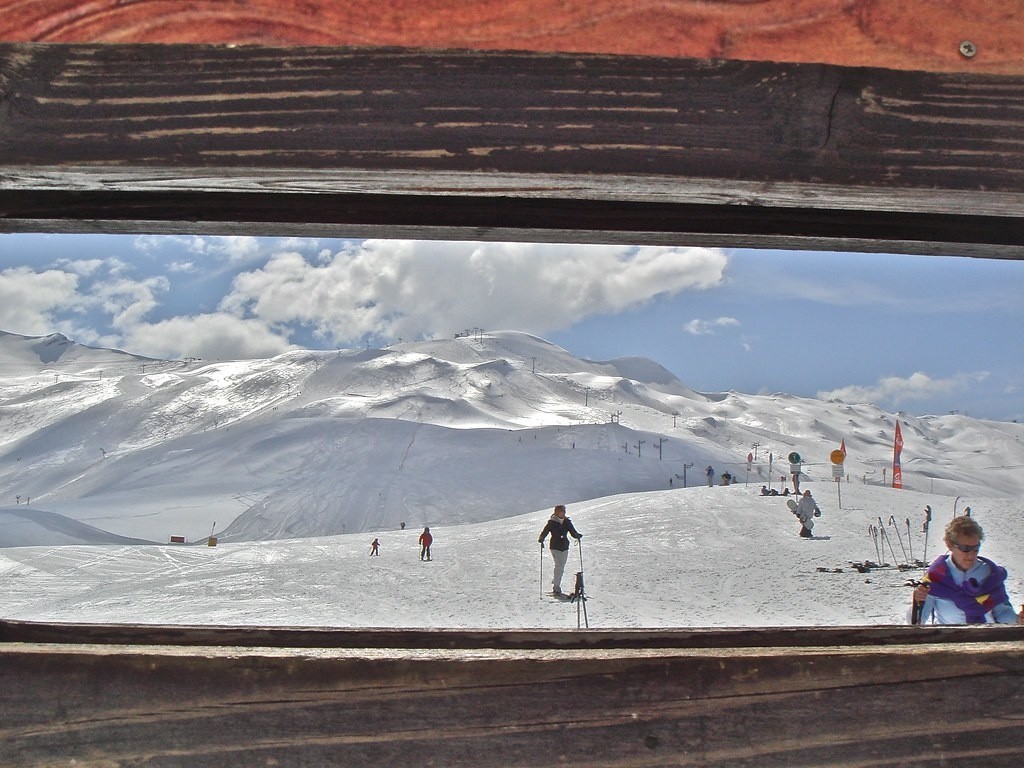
[545,592,586,602]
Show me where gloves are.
[538,539,543,543]
[577,534,583,538]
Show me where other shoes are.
[553,586,561,594]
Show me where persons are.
[762,483,801,496]
[798,490,821,537]
[370,539,381,556]
[907,515,1023,627]
[400,522,406,530]
[722,470,731,485]
[538,505,582,594]
[706,466,714,487]
[418,527,433,562]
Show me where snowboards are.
[786,500,814,530]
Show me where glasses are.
[950,540,980,552]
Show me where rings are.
[918,594,921,598]
